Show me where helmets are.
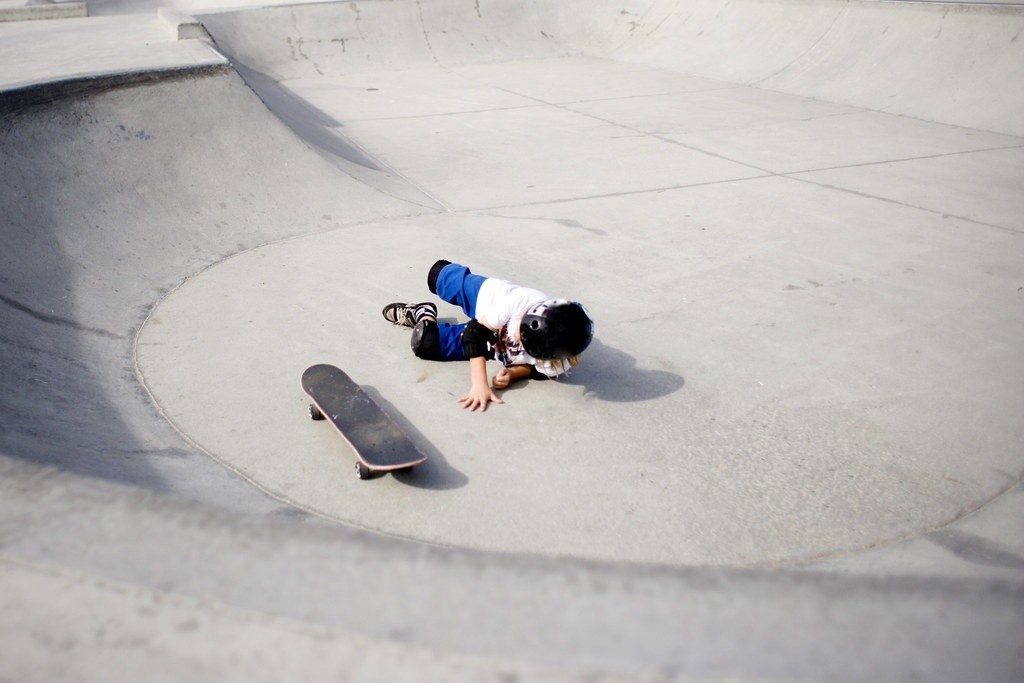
[520,298,594,361]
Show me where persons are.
[383,259,595,412]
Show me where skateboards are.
[299,363,428,479]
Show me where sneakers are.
[381,302,437,327]
